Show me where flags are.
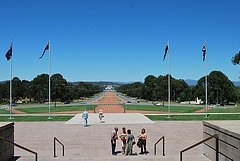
[5,43,12,60]
[163,40,168,62]
[39,41,50,59]
[202,46,206,61]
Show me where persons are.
[111,127,118,155]
[98,109,103,120]
[82,109,88,127]
[137,128,147,154]
[120,127,135,155]
